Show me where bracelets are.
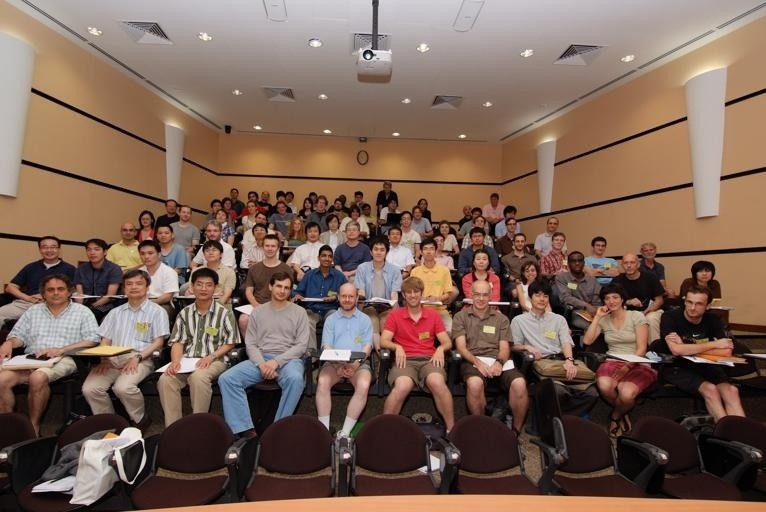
[358,358,363,364]
[211,352,216,360]
[135,353,143,362]
[496,359,505,365]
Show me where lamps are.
[0,31,37,197]
[682,65,725,219]
[536,139,556,214]
[164,123,186,201]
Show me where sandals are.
[610,416,622,437]
[621,413,631,432]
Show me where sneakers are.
[335,430,352,454]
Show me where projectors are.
[356,49,392,84]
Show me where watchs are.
[566,357,574,362]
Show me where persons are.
[315,282,374,454]
[658,284,746,425]
[582,283,659,438]
[218,271,311,439]
[0,181,272,329]
[81,269,170,435]
[380,276,454,437]
[156,268,236,430]
[452,280,530,439]
[236,181,460,350]
[510,280,599,417]
[456,193,721,351]
[0,273,101,438]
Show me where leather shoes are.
[131,413,152,432]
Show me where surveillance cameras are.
[224,125,232,134]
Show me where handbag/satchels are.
[485,396,513,431]
[69,427,146,506]
[56,396,93,435]
[533,359,595,383]
[293,266,311,285]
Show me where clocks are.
[357,150,369,166]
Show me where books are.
[695,354,748,364]
